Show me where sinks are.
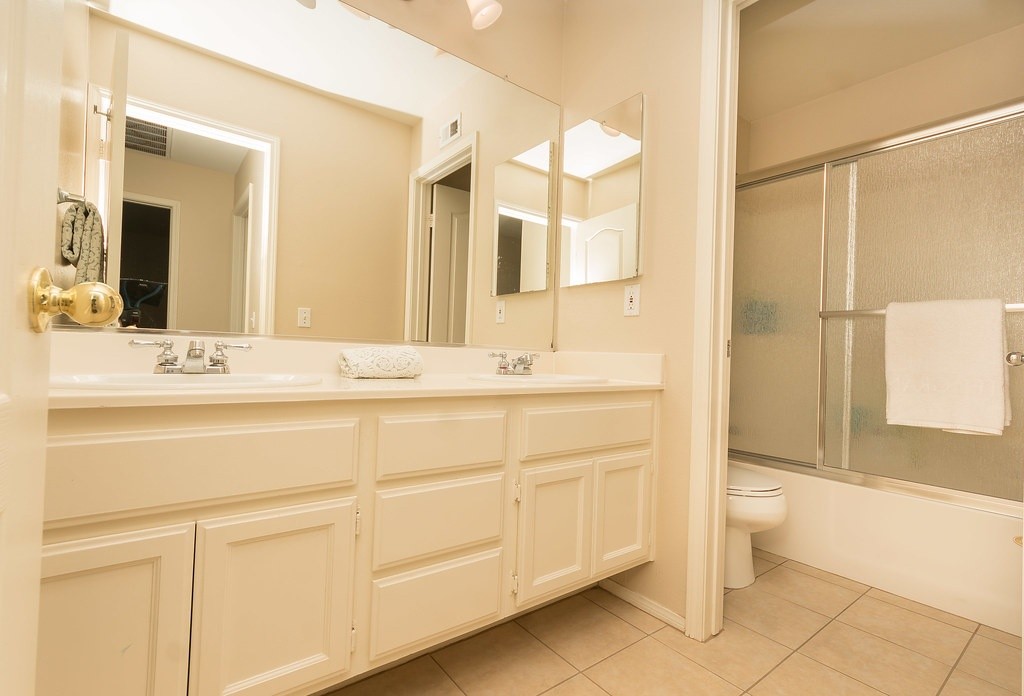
[445,373,609,384]
[47,370,323,392]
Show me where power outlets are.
[624,284,639,317]
[496,300,506,324]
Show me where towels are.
[882,297,1014,437]
[337,345,424,379]
[59,200,104,287]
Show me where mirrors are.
[560,93,645,283]
[492,139,554,297]
[49,0,562,351]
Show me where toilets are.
[724,463,789,590]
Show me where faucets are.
[180,340,206,374]
[510,352,532,370]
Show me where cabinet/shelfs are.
[36,392,658,696]
